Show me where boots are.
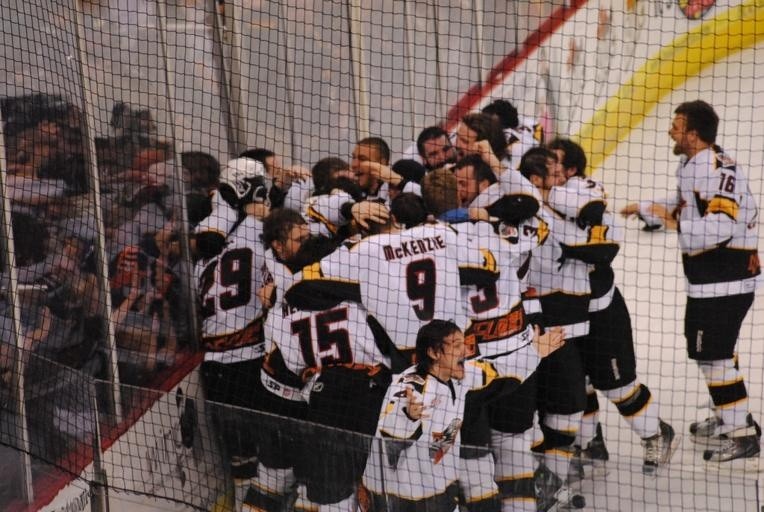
[581,420,609,463]
[533,464,571,510]
[565,445,584,485]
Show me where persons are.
[0,87,223,470]
[186,97,678,510]
[618,96,764,466]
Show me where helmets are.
[217,157,273,204]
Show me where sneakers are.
[688,411,760,464]
[641,416,675,478]
